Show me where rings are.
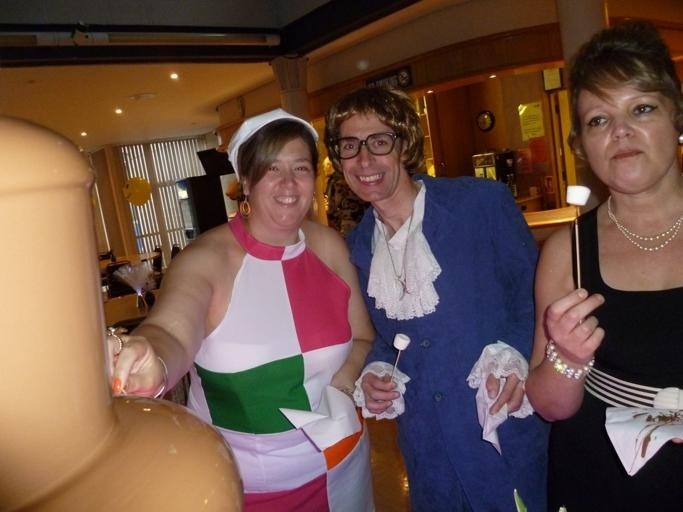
[108,327,123,357]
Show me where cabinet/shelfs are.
[408,87,475,180]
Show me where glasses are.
[330,132,398,161]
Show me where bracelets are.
[541,340,594,382]
[157,355,168,402]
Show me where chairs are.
[153,248,163,270]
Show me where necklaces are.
[380,181,416,299]
[606,196,683,253]
[605,194,680,241]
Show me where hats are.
[225,108,319,184]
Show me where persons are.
[322,82,549,511]
[108,108,378,509]
[520,16,683,511]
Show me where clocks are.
[476,111,495,130]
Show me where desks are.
[99,252,162,330]
